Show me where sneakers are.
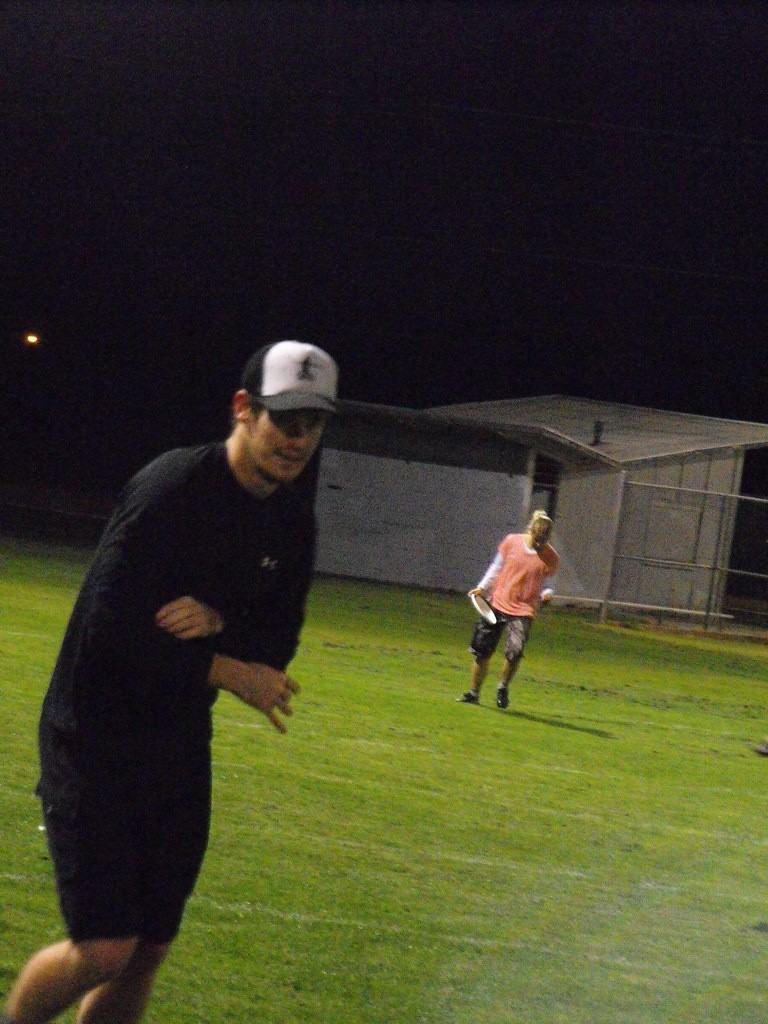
[497,688,508,707]
[457,693,479,705]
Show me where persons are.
[0,340,342,1024]
[456,510,561,708]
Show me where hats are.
[242,341,338,414]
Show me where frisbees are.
[470,593,497,625]
